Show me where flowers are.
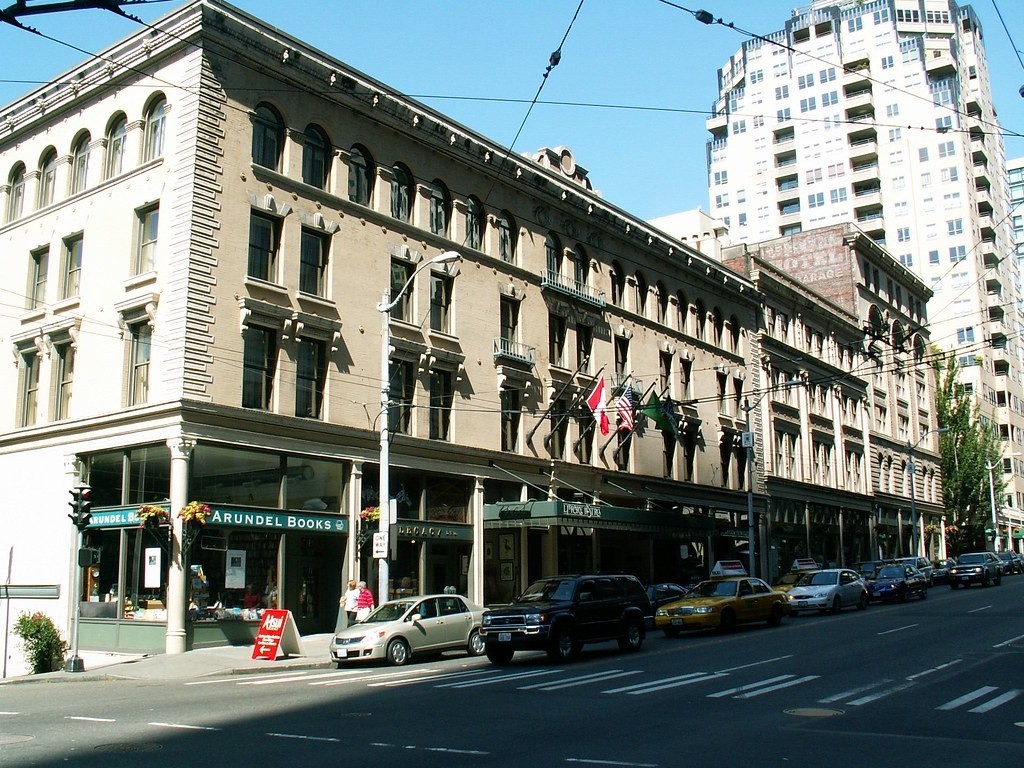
[175,502,209,521]
[359,507,382,521]
[134,507,167,528]
[925,525,936,533]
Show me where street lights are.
[379,252,460,607]
[747,380,805,576]
[907,428,949,556]
[989,452,1022,553]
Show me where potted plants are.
[12,610,67,673]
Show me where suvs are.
[478,575,654,666]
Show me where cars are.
[642,584,696,610]
[787,568,869,614]
[329,595,490,667]
[949,552,1024,589]
[772,571,811,592]
[656,578,792,637]
[851,556,956,604]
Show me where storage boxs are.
[147,600,165,609]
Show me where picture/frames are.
[484,542,492,562]
[461,555,469,576]
[501,563,514,581]
[498,535,514,560]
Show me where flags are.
[614,379,634,431]
[640,390,680,441]
[586,373,610,436]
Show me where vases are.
[928,528,934,533]
[367,519,380,531]
[184,518,204,531]
[143,516,159,528]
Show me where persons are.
[344,580,360,630]
[244,584,263,612]
[358,581,374,622]
[398,577,411,599]
[407,606,420,621]
